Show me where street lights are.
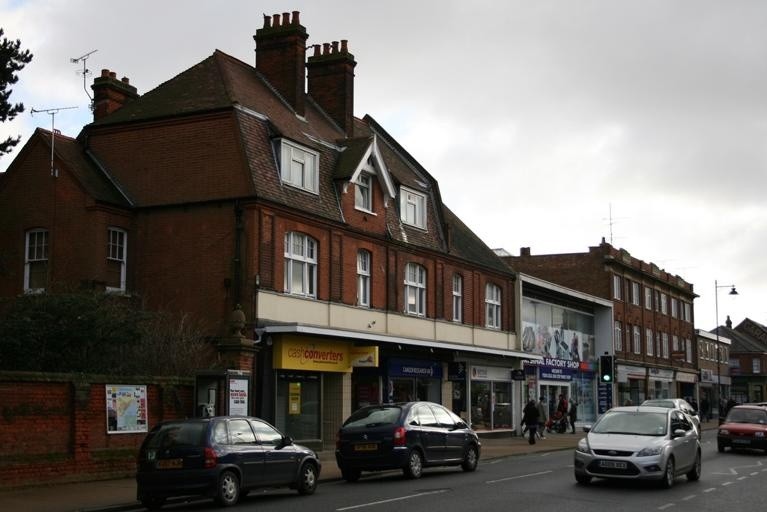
[714,279,739,410]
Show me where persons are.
[686,395,736,422]
[520,395,579,443]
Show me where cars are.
[331,398,485,479]
[639,396,703,442]
[714,404,767,457]
[571,405,707,495]
[129,413,322,503]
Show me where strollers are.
[546,411,569,433]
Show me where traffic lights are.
[612,356,618,383]
[600,355,614,384]
